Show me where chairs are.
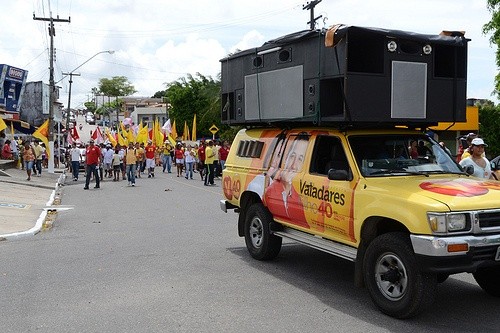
[331,144,349,171]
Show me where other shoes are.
[135,169,220,181]
[204,182,211,186]
[33,173,37,175]
[105,174,107,177]
[27,178,30,180]
[211,182,216,185]
[73,178,77,181]
[132,183,135,186]
[113,178,116,181]
[129,181,131,185]
[37,174,41,176]
[108,175,111,178]
[84,187,89,190]
[123,177,126,180]
[94,186,100,188]
[117,177,119,181]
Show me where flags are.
[0,116,7,131]
[32,118,49,159]
[66,114,198,148]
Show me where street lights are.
[48,50,115,173]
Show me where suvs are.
[219,125,500,320]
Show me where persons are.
[246,131,310,229]
[0,129,59,168]
[408,133,495,180]
[31,140,42,177]
[69,142,83,181]
[80,124,82,130]
[175,135,230,186]
[20,141,37,180]
[163,140,173,173]
[83,138,103,190]
[81,144,164,181]
[123,142,139,186]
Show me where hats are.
[148,139,152,143]
[164,140,170,143]
[470,138,488,147]
[89,139,94,143]
[466,133,476,141]
